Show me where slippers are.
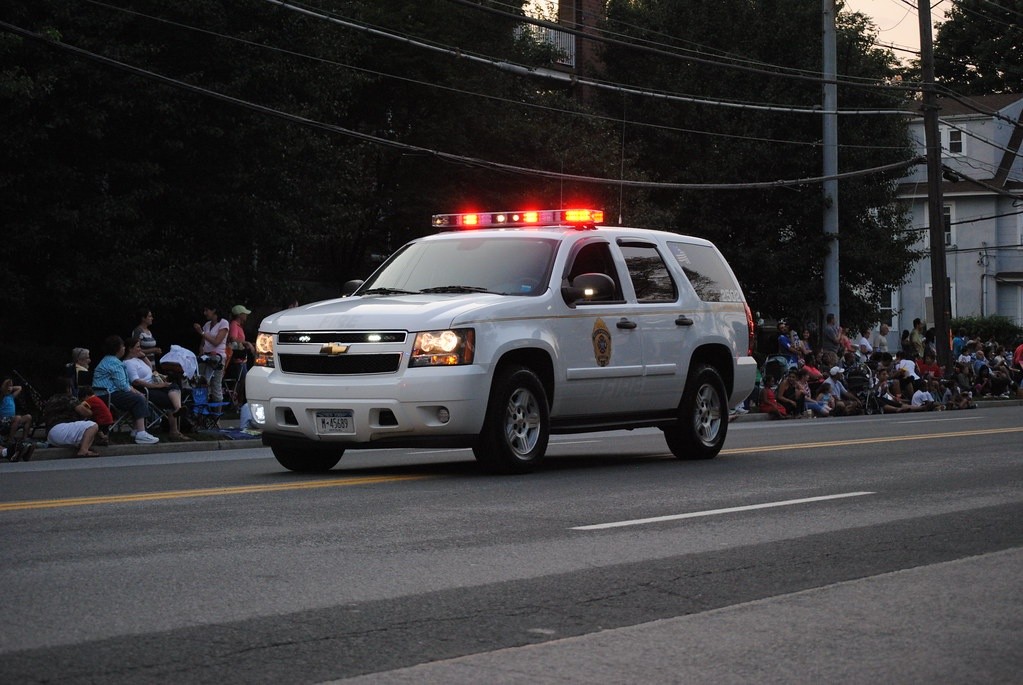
[173,406,188,418]
[169,433,193,441]
[76,452,100,457]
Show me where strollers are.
[842,358,884,415]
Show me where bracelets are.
[201,331,204,335]
[142,356,145,358]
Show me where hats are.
[232,305,251,315]
[830,366,844,375]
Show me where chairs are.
[14,350,264,446]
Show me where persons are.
[0,298,299,463]
[729,313,1023,419]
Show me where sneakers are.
[135,432,159,444]
[131,430,137,437]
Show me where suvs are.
[244,208,754,478]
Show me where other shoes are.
[20,442,35,462]
[999,394,1009,398]
[7,440,22,462]
[737,407,749,415]
